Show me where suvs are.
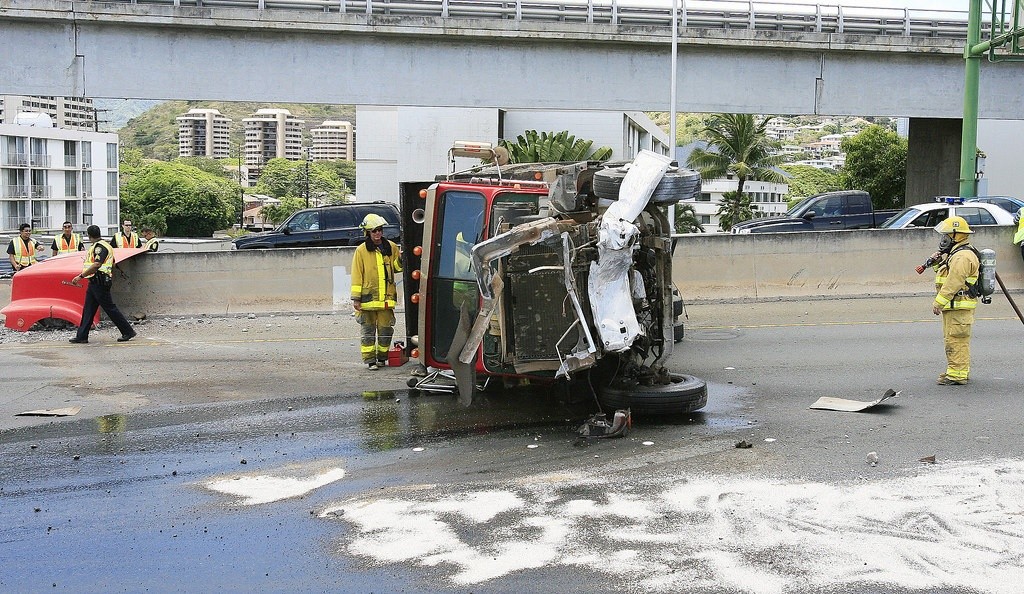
[231,200,403,250]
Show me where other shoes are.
[377,359,384,367]
[935,372,957,385]
[69,337,88,344]
[368,363,378,370]
[118,331,137,342]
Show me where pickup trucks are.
[730,189,906,235]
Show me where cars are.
[876,196,1017,228]
[966,196,1024,217]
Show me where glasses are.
[145,232,149,237]
[21,229,32,232]
[124,224,132,227]
[64,226,71,229]
[370,227,383,234]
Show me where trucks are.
[387,140,708,420]
[140,236,240,250]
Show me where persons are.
[309,214,319,230]
[140,226,159,252]
[68,226,136,343]
[5,223,45,274]
[931,216,979,385]
[452,231,480,312]
[110,219,142,248]
[1012,206,1024,264]
[350,213,404,370]
[50,222,85,257]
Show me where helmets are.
[359,213,388,232]
[941,216,974,234]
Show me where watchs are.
[78,274,84,278]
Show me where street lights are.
[304,146,314,209]
